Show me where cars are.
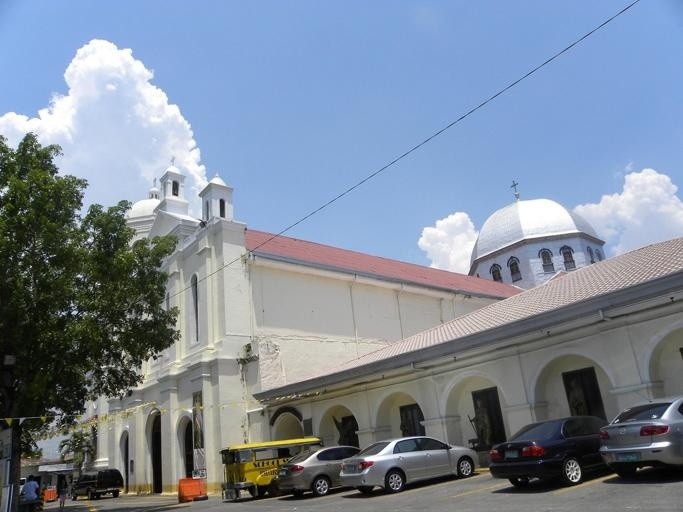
[487,415,609,489]
[597,395,683,479]
[339,435,480,494]
[275,445,362,497]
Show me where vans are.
[70,468,125,500]
[219,435,323,502]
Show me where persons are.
[471,399,492,445]
[20,474,40,512]
[58,478,68,508]
[569,378,588,416]
